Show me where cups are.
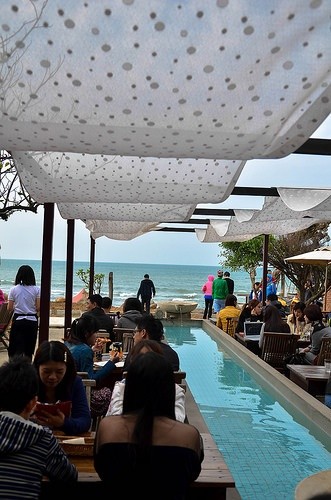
[324,359,331,373]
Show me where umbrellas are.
[283,246,331,312]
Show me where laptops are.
[244,322,265,341]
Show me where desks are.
[0,302,331,499]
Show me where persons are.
[92,351,205,500]
[211,270,228,314]
[80,294,115,353]
[0,354,80,500]
[121,315,180,376]
[235,269,331,379]
[63,315,121,381]
[136,274,156,314]
[222,271,234,295]
[31,340,91,435]
[117,297,146,330]
[201,275,215,319]
[100,297,112,313]
[108,340,187,424]
[217,295,242,338]
[6,264,41,361]
